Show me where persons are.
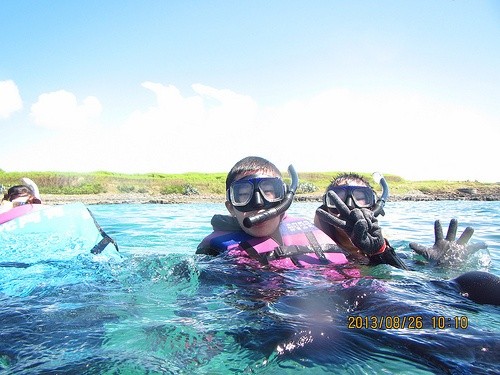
[313,172,500,309]
[194,155,500,375]
[0,185,41,225]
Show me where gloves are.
[409,218,489,269]
[327,190,386,255]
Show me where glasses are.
[229,177,286,213]
[325,185,376,210]
[15,187,33,197]
